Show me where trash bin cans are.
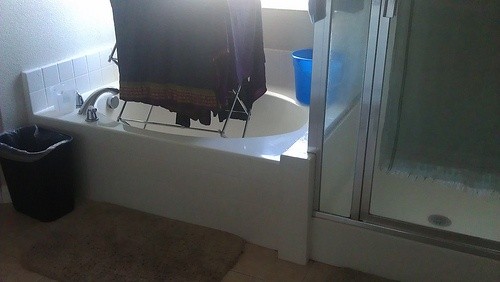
[0,124,78,224]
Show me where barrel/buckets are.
[291,49,347,107]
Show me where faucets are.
[78,87,119,115]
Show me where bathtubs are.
[34,75,310,251]
